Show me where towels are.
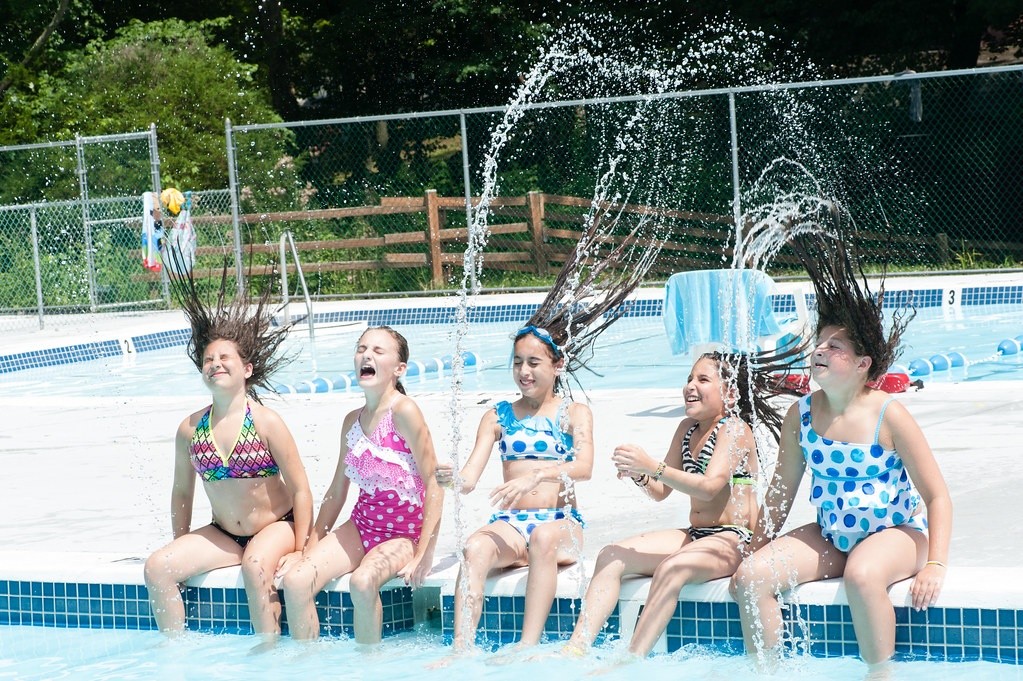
[662,267,807,374]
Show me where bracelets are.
[926,561,950,570]
[631,473,646,482]
[651,461,667,482]
[635,476,649,487]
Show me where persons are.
[569,321,818,659]
[433,190,674,650]
[282,325,445,644]
[728,207,955,672]
[144,235,323,634]
